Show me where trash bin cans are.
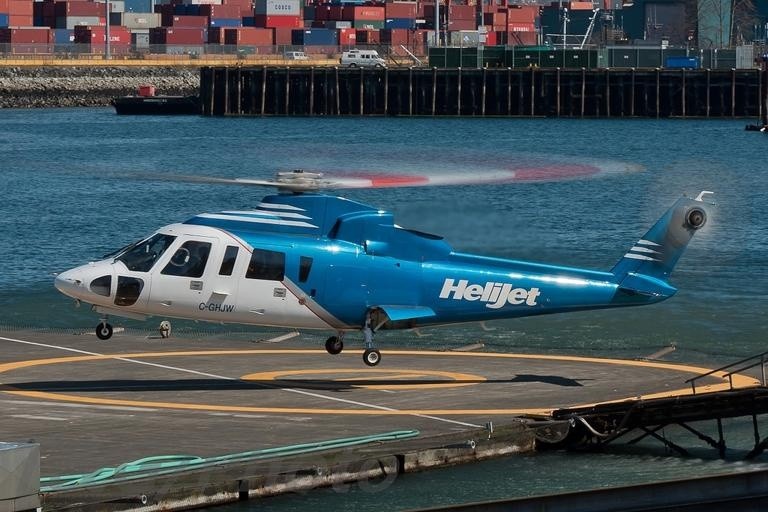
[138,84,155,97]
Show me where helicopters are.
[50,155,742,368]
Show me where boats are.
[108,93,201,115]
[745,115,768,132]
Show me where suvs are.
[286,51,309,60]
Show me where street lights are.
[714,48,718,69]
[563,7,568,50]
[701,49,704,69]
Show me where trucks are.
[339,49,387,68]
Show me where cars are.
[754,51,768,62]
